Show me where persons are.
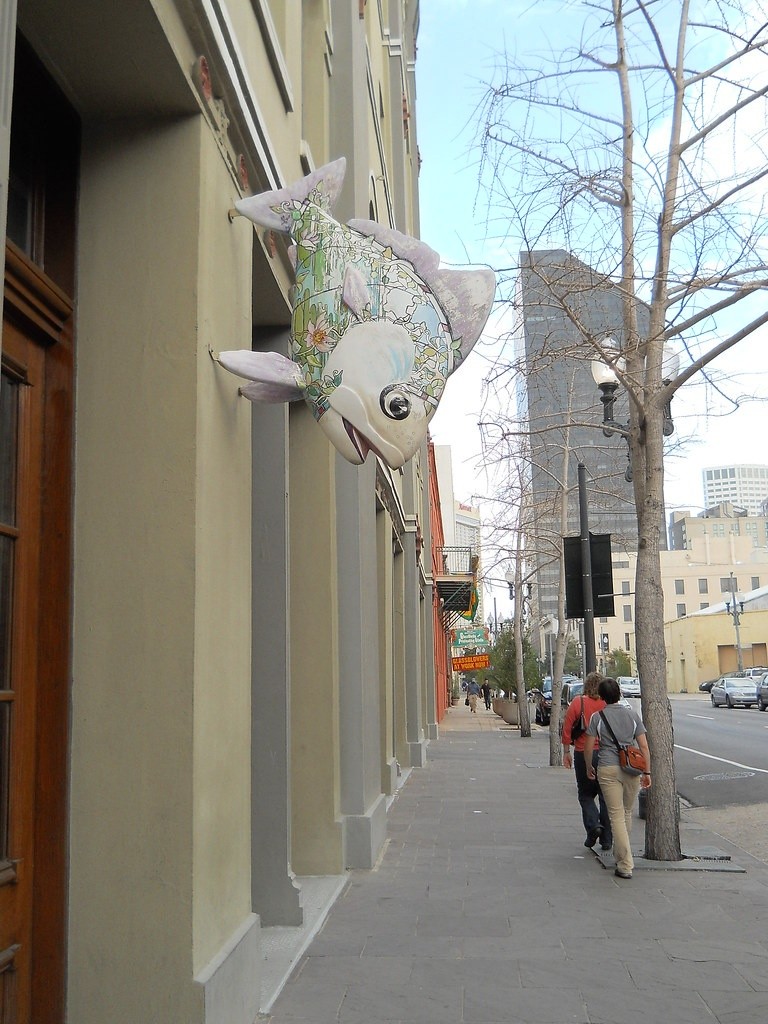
[561,673,613,850]
[481,679,491,710]
[466,678,479,714]
[584,677,652,879]
[461,681,471,692]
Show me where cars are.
[615,676,641,698]
[699,670,744,694]
[711,678,757,709]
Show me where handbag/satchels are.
[619,746,647,775]
[465,699,469,706]
[571,695,587,739]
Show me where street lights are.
[725,588,745,671]
[488,612,505,699]
[597,635,609,676]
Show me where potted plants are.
[488,624,545,726]
[452,694,460,705]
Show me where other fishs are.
[217,155,496,472]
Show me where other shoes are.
[584,828,602,847]
[615,868,632,879]
[602,846,610,850]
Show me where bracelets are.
[564,751,570,753]
[643,772,651,775]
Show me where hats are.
[471,678,476,683]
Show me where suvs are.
[756,672,768,711]
[532,675,578,727]
[559,679,588,740]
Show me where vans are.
[745,666,768,685]
